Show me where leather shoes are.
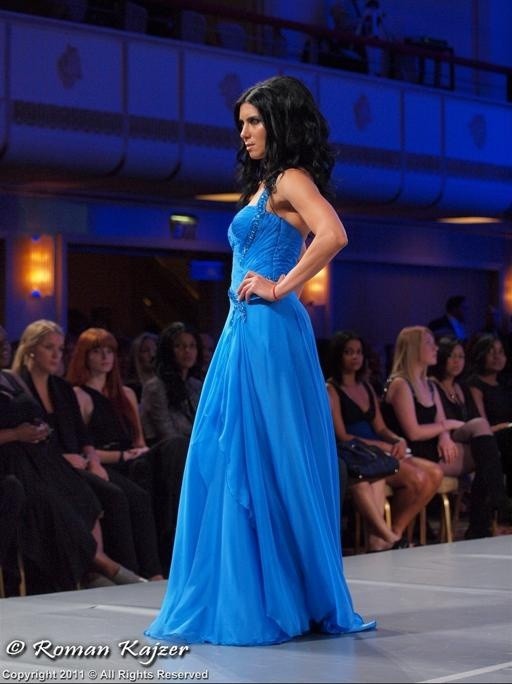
[86,563,149,587]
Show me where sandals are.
[366,538,410,553]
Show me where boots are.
[466,435,512,539]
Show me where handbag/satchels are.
[337,439,399,480]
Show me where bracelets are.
[272,282,279,302]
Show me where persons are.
[143,74,380,648]
[0,320,512,597]
[428,295,469,342]
[483,302,502,329]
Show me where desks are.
[403,34,456,90]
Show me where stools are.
[1,444,505,598]
